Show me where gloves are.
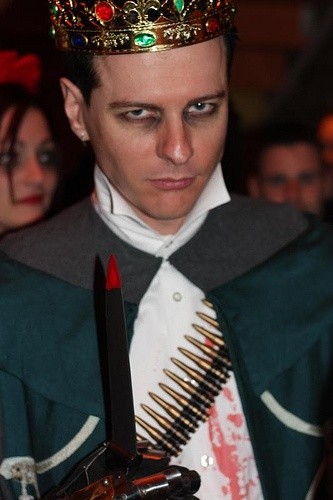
[40,439,203,499]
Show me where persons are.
[0,0,333,500]
[0,52,59,238]
[246,110,333,226]
